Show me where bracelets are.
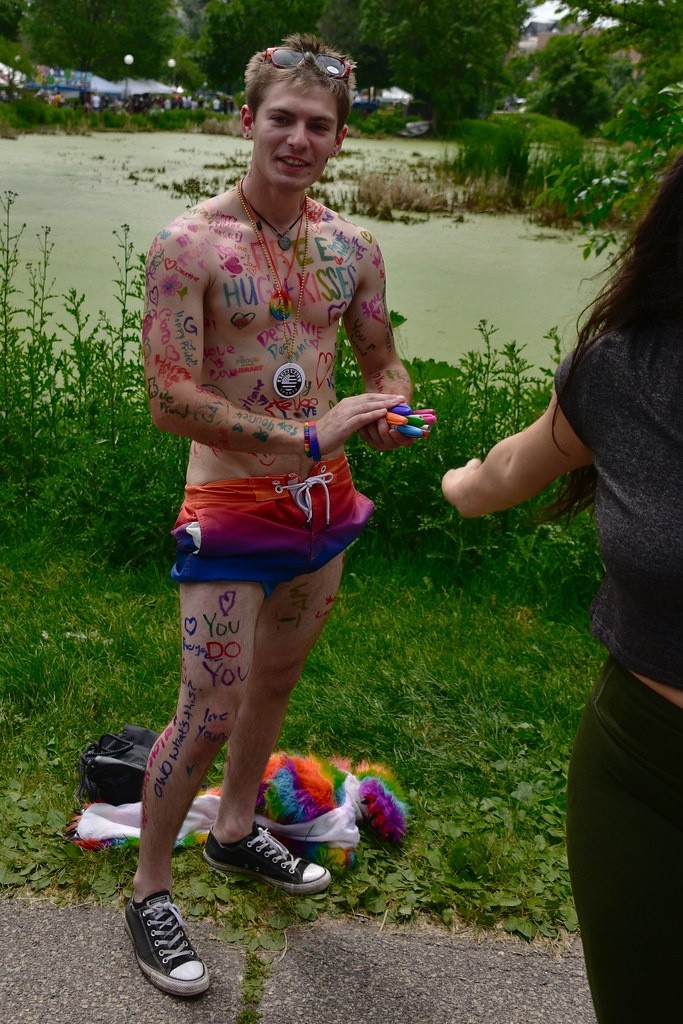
[304,420,321,461]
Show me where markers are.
[386,404,436,439]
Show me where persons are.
[441,150,683,1024]
[45,88,234,114]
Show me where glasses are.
[264,48,351,81]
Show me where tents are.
[351,86,413,107]
[26,68,173,95]
[124,32,416,996]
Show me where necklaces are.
[237,177,309,399]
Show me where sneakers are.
[203,824,331,894]
[123,891,208,994]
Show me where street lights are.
[123,54,134,107]
[168,58,177,94]
